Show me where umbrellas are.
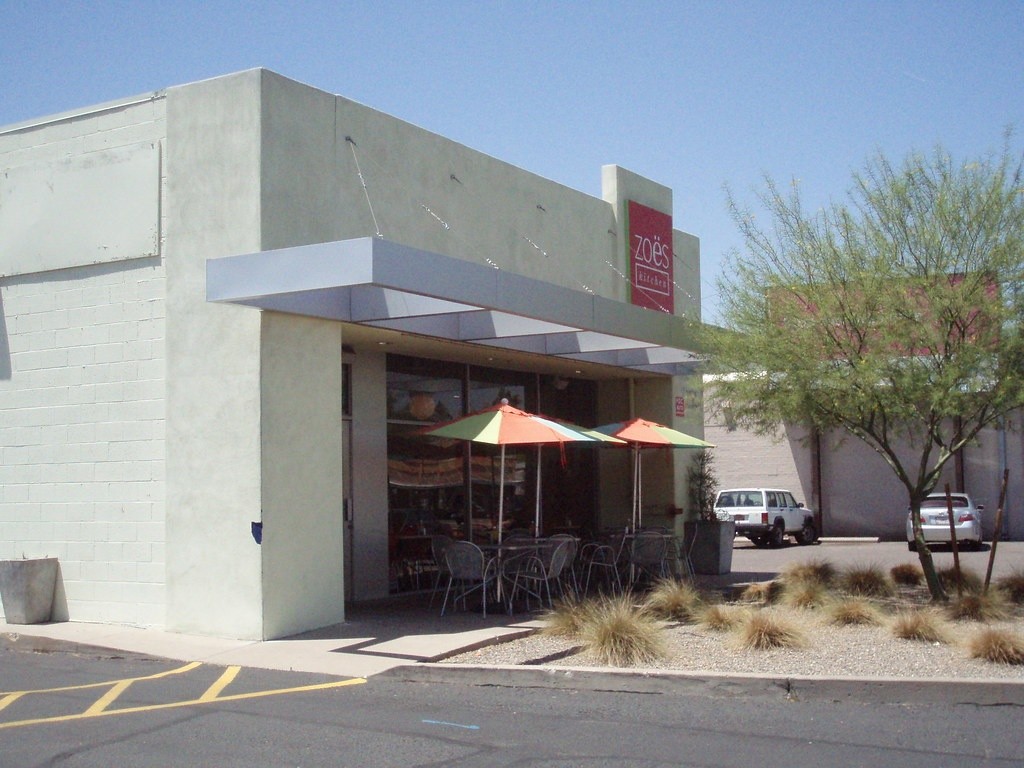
[407,398,631,603]
[589,418,714,527]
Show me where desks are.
[468,544,552,612]
[511,537,581,542]
[609,532,675,539]
[554,525,581,529]
[396,534,430,539]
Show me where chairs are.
[743,498,755,505]
[388,519,696,620]
[722,496,734,507]
[769,498,777,506]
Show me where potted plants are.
[0,553,58,625]
[685,448,735,575]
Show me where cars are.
[905,493,986,551]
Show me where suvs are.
[712,486,816,550]
